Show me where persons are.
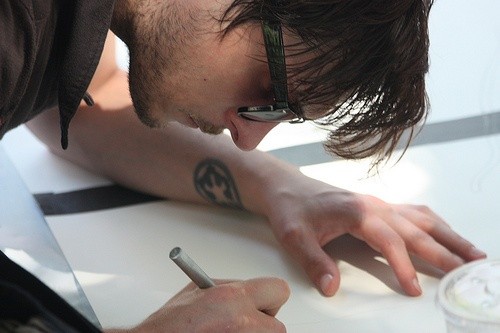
[1,0,488,331]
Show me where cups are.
[435,255,500,333]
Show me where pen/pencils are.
[168,246,214,289]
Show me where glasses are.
[236,18,306,125]
